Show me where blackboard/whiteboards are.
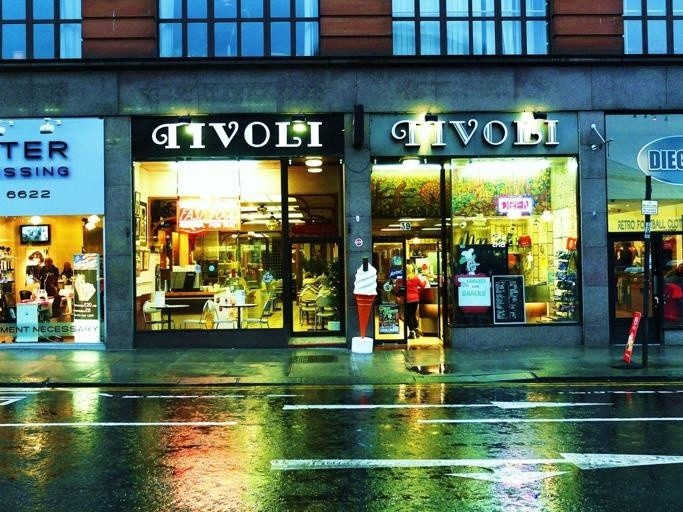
[377,301,403,337]
[492,275,527,324]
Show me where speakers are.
[352,103,365,149]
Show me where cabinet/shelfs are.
[16,296,74,343]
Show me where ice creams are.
[353,262,378,339]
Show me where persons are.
[26,251,43,282]
[39,258,59,289]
[60,262,73,279]
[394,264,426,339]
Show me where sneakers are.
[405,329,424,339]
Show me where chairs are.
[299,283,338,331]
[143,279,283,330]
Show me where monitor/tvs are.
[20,224,51,245]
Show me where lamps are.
[179,116,191,125]
[0,120,14,136]
[425,115,439,122]
[39,117,60,134]
[291,114,306,122]
[534,113,548,120]
